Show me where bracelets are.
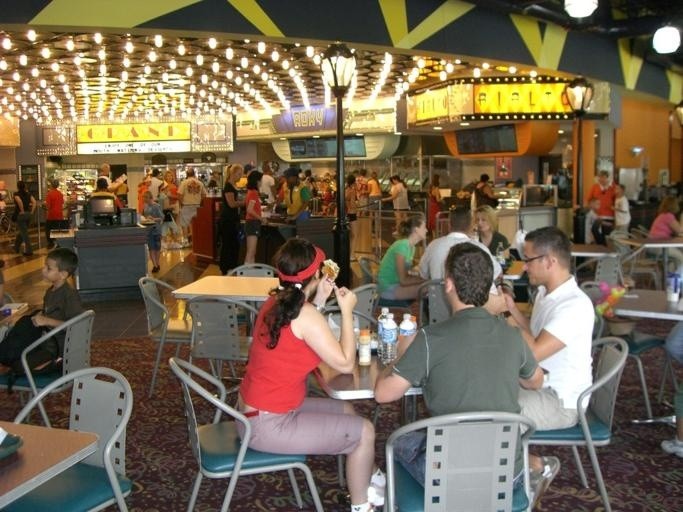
[310,300,327,317]
[493,310,512,322]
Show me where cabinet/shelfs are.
[492,186,523,248]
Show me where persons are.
[375,216,432,302]
[482,225,594,496]
[423,171,500,241]
[41,179,64,248]
[94,163,125,227]
[584,170,682,247]
[143,165,223,272]
[659,321,683,460]
[232,235,384,512]
[220,160,338,275]
[1,249,88,379]
[0,180,36,257]
[373,242,544,496]
[344,169,409,235]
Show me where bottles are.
[399,313,414,336]
[357,330,371,367]
[495,241,505,269]
[376,306,388,358]
[381,314,397,365]
[409,315,416,330]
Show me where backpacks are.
[0,309,58,395]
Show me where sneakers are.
[367,482,385,505]
[152,265,160,273]
[661,439,683,458]
[529,455,560,511]
[13,246,19,253]
[24,252,32,256]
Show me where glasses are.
[524,255,543,262]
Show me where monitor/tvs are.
[87,197,114,213]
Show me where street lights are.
[564,77,595,245]
[318,43,358,287]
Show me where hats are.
[244,164,256,171]
[278,244,325,282]
[284,168,301,177]
